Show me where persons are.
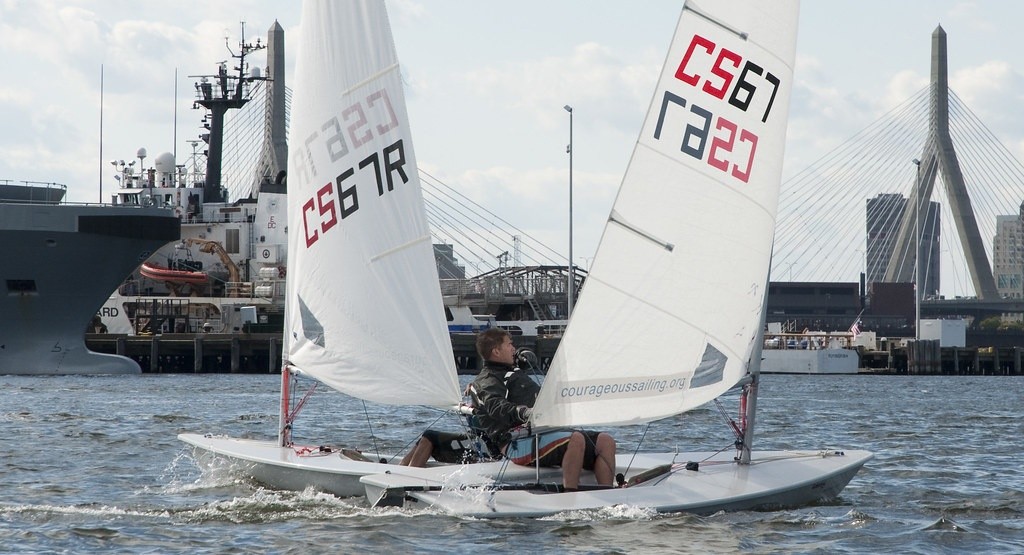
[126,275,134,296]
[399,384,502,468]
[471,329,616,491]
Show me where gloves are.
[517,350,537,370]
[523,407,533,421]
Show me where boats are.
[0,64,183,378]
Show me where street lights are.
[913,158,921,340]
[564,104,573,317]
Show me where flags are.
[851,320,860,336]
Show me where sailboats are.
[175,1,871,524]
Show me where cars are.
[764,339,808,350]
